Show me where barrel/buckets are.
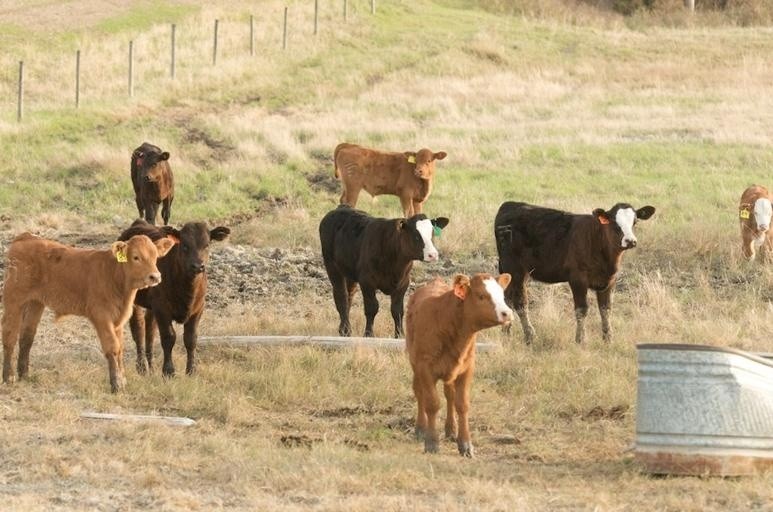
[637,343,769,477]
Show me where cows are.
[116,217,231,380]
[406,273,514,459]
[494,201,656,346]
[738,183,773,268]
[318,207,450,339]
[0,232,176,395]
[130,142,174,227]
[334,142,447,220]
